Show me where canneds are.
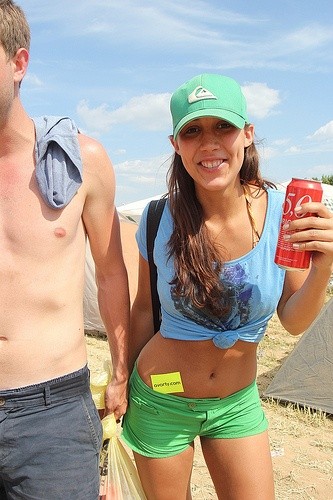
[273,177,323,271]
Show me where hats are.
[170,73,248,141]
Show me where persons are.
[109,73,333,500]
[0,0,130,499]
